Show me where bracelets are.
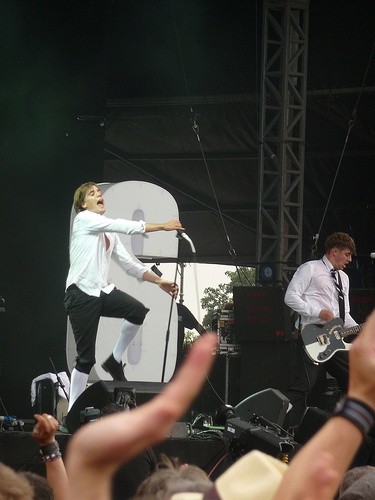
[330,397,375,439]
[39,440,62,462]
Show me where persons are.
[279,231,361,443]
[63,181,186,414]
[0,311,375,500]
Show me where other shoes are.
[100,355,128,382]
[59,418,72,433]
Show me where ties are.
[328,268,345,325]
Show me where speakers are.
[239,343,315,432]
[236,389,289,437]
[65,380,169,433]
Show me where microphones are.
[176,229,196,253]
